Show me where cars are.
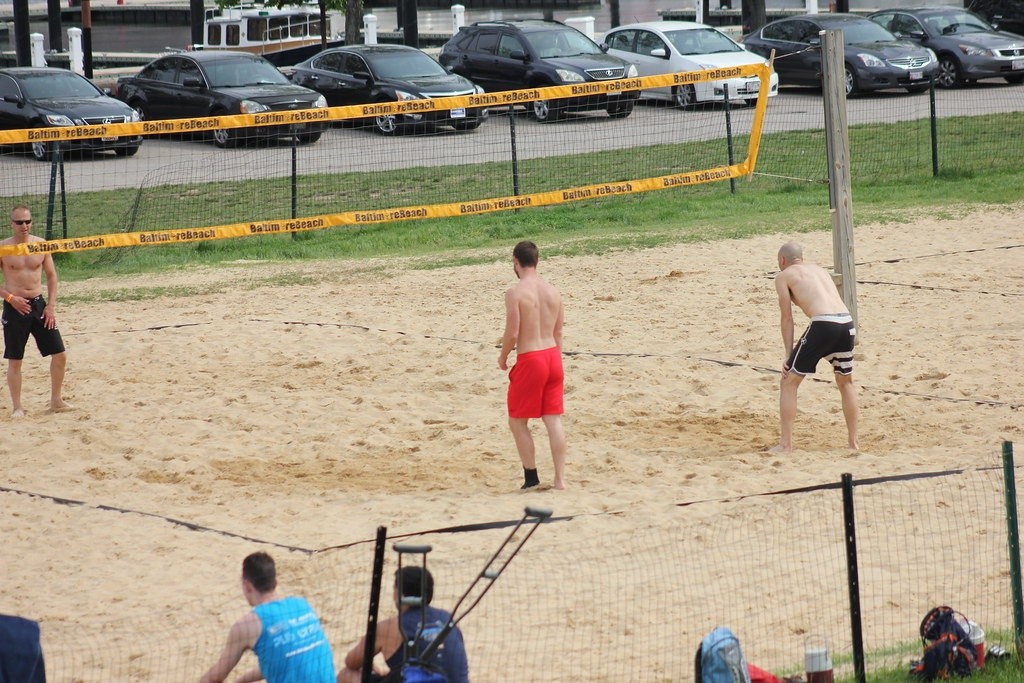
[0,66,143,162]
[960,0,1024,33]
[588,21,779,111]
[864,7,1024,91]
[114,51,332,151]
[291,44,489,137]
[736,13,941,99]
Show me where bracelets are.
[7,294,13,302]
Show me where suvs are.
[439,19,642,124]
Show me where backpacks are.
[695,626,751,683]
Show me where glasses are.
[10,218,33,225]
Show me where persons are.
[497,241,566,489]
[336,566,469,683]
[199,552,336,683]
[768,242,860,451]
[0,206,73,417]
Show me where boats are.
[200,4,333,53]
